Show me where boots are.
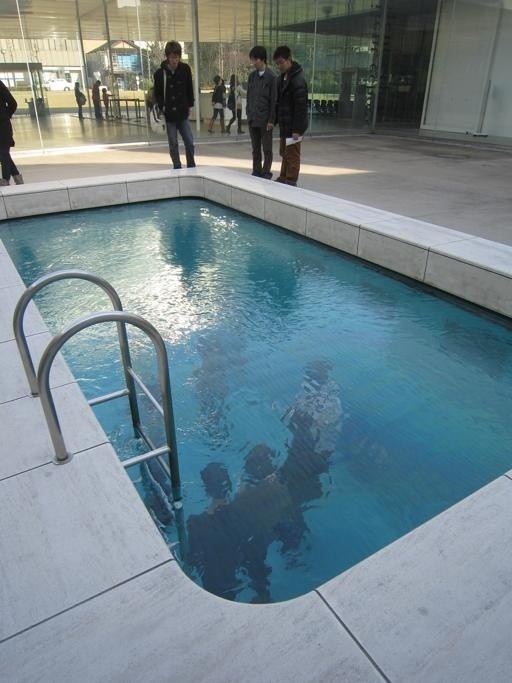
[220,119,226,133]
[1,173,24,185]
[207,116,216,133]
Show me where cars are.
[45,79,76,91]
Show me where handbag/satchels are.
[147,102,167,137]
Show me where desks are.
[110,97,146,121]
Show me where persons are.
[281,413,334,510]
[206,74,228,133]
[244,44,279,179]
[225,444,300,602]
[1,78,27,192]
[74,81,86,119]
[223,73,249,134]
[271,44,309,187]
[294,357,347,468]
[92,79,104,120]
[102,87,115,120]
[150,39,197,167]
[185,461,251,602]
